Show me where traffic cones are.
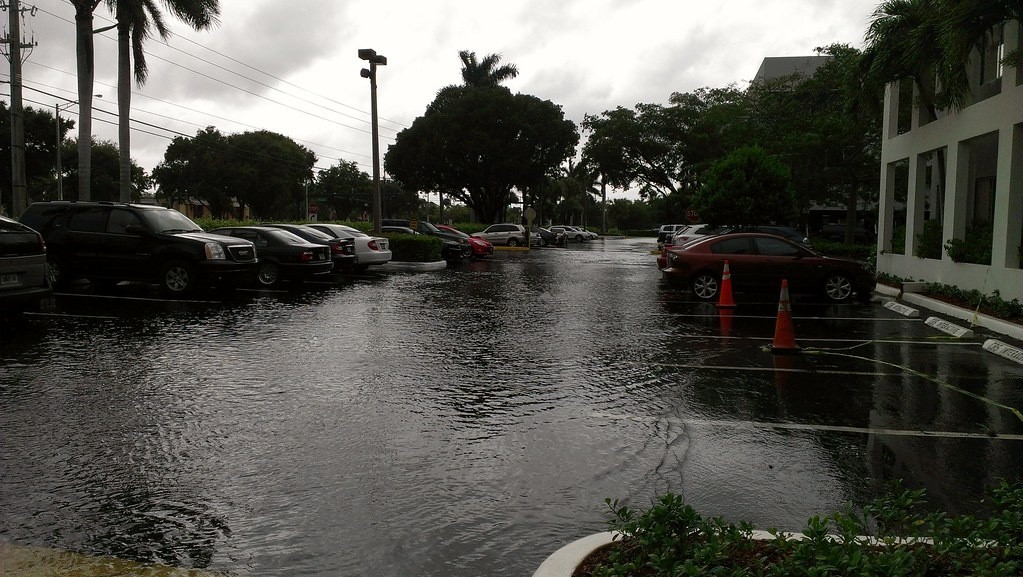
[765,278,801,353]
[715,259,737,308]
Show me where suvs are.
[19,199,258,299]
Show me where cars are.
[0,215,55,326]
[546,225,599,245]
[662,233,877,303]
[469,223,541,248]
[523,225,558,247]
[260,224,358,276]
[657,224,812,273]
[302,224,394,275]
[203,226,334,290]
[370,219,495,268]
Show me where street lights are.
[358,48,387,236]
[56,94,103,198]
